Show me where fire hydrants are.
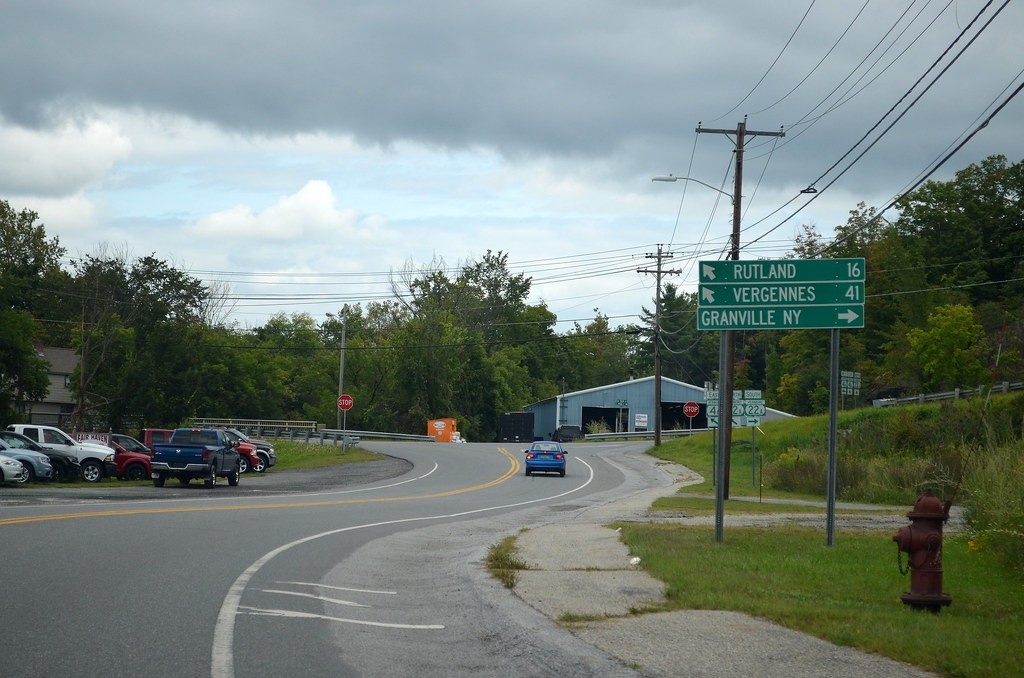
[891,494,952,615]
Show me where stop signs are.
[683,401,700,417]
[337,395,353,411]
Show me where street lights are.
[326,313,345,429]
[652,175,742,499]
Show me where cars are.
[557,425,581,443]
[66,434,151,483]
[0,455,24,487]
[524,442,569,477]
[0,438,53,485]
[0,430,82,483]
[143,427,277,475]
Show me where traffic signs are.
[698,260,865,330]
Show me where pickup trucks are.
[7,424,118,484]
[150,429,242,490]
